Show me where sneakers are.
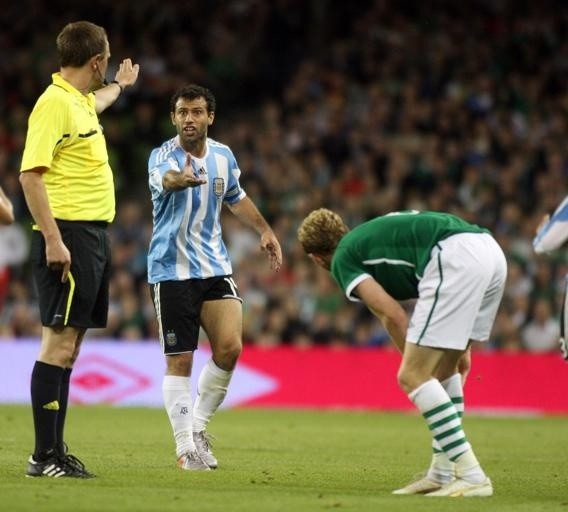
[193,428,220,472]
[172,449,211,475]
[22,442,100,481]
[388,476,458,496]
[422,468,496,504]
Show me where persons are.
[147,88,284,470]
[0,1,568,359]
[296,208,508,499]
[18,20,139,478]
